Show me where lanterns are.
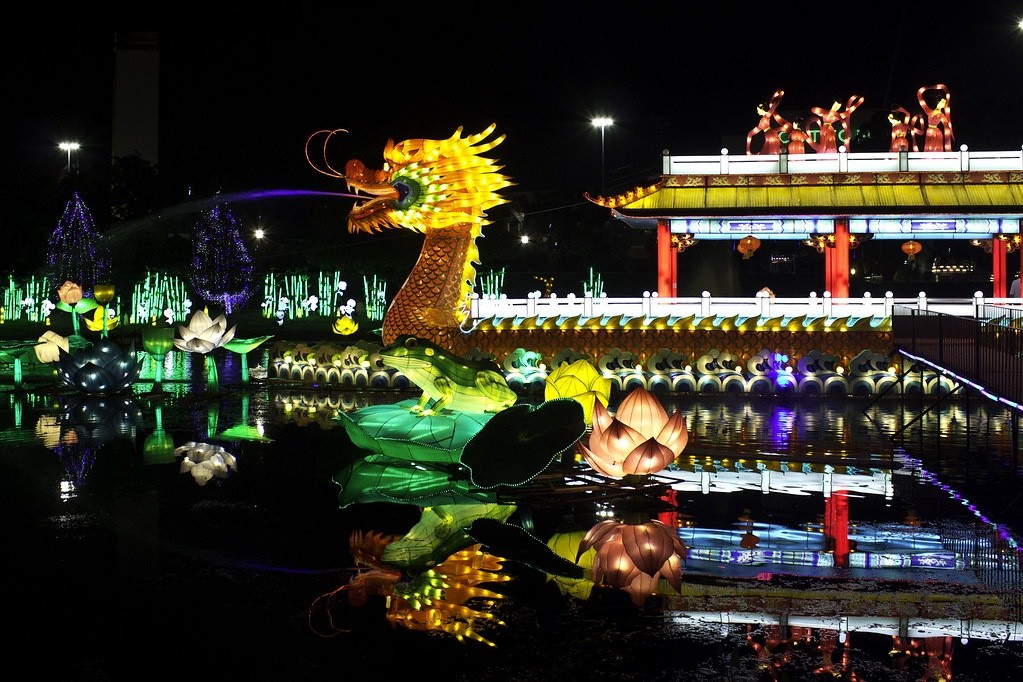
[901,241,922,264]
[737,236,760,259]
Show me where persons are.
[1009,276,1021,305]
